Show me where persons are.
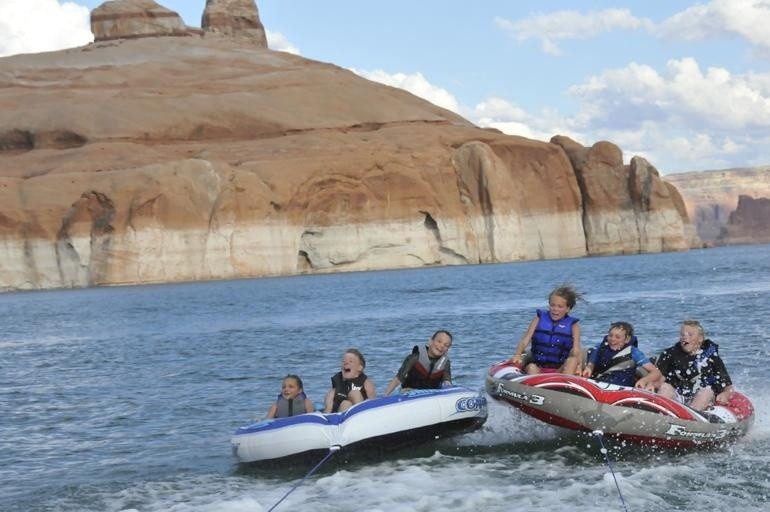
[512,285,580,376]
[586,323,661,388]
[324,349,377,413]
[647,319,735,412]
[384,329,452,394]
[264,374,313,420]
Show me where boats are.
[230,385,489,468]
[484,356,755,449]
